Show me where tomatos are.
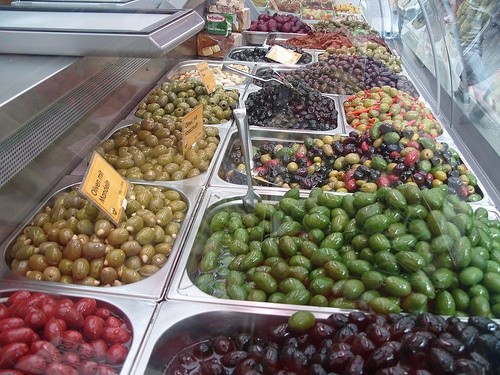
[0,290,131,375]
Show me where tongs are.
[223,60,302,96]
[232,108,262,213]
